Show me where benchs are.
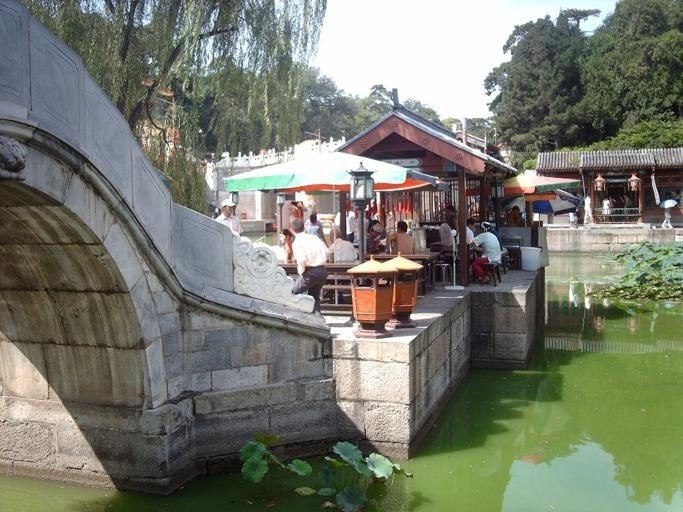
[501,254,511,274]
[481,261,501,287]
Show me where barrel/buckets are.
[519,246,542,272]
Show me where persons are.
[583,193,595,224]
[662,208,673,229]
[601,193,634,222]
[569,212,578,226]
[215,199,244,233]
[280,201,524,313]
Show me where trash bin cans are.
[347,254,399,339]
[381,251,425,329]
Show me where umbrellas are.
[224,152,440,304]
[661,200,678,208]
[503,169,581,228]
[495,188,580,227]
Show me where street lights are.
[276,193,284,231]
[347,161,375,285]
[227,190,239,217]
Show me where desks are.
[276,242,476,322]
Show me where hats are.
[222,200,235,208]
